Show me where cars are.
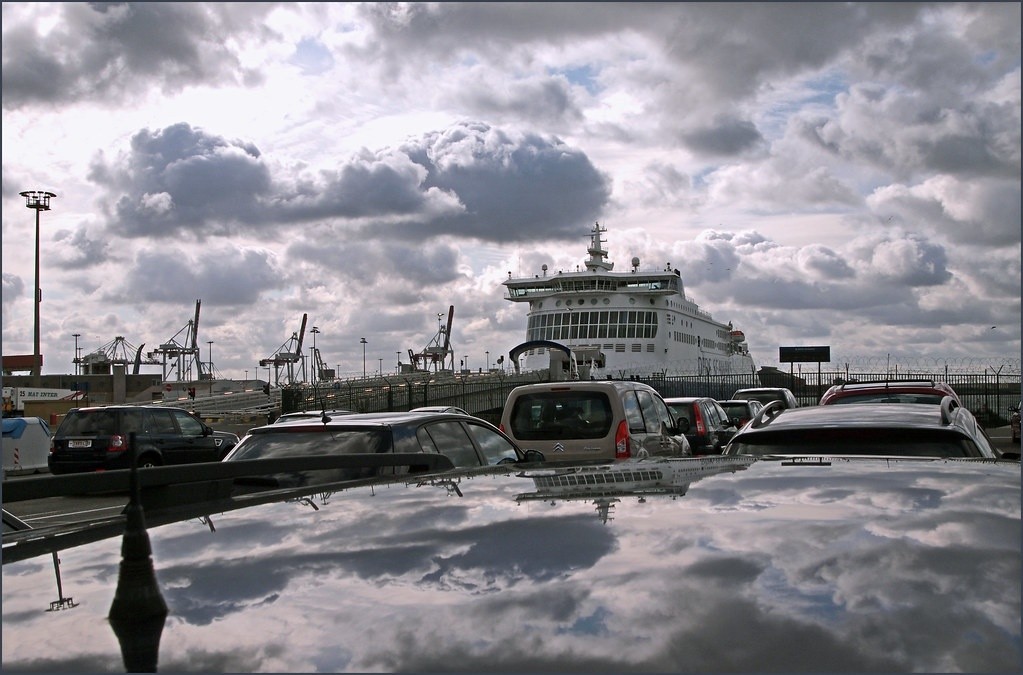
[220,411,547,495]
[1007,401,1022,443]
[411,406,469,415]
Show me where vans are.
[663,397,739,454]
[500,381,693,466]
[727,388,801,418]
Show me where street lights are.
[397,351,401,372]
[485,351,490,371]
[72,334,83,407]
[207,340,214,393]
[20,190,56,389]
[359,338,368,382]
[310,327,320,386]
[378,359,383,375]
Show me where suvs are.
[48,405,241,476]
[717,398,765,425]
[817,379,963,408]
[724,400,1020,461]
[274,408,358,422]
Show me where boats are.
[501,219,761,395]
